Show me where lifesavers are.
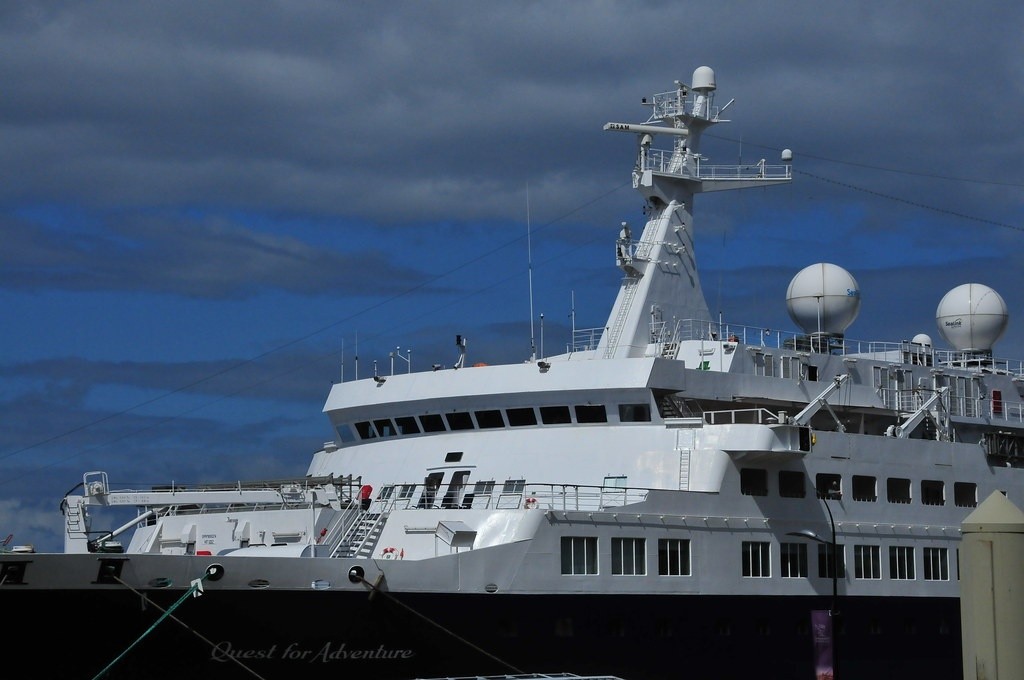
[380,547,399,559]
[524,498,539,508]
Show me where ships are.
[0,62,1024,680]
